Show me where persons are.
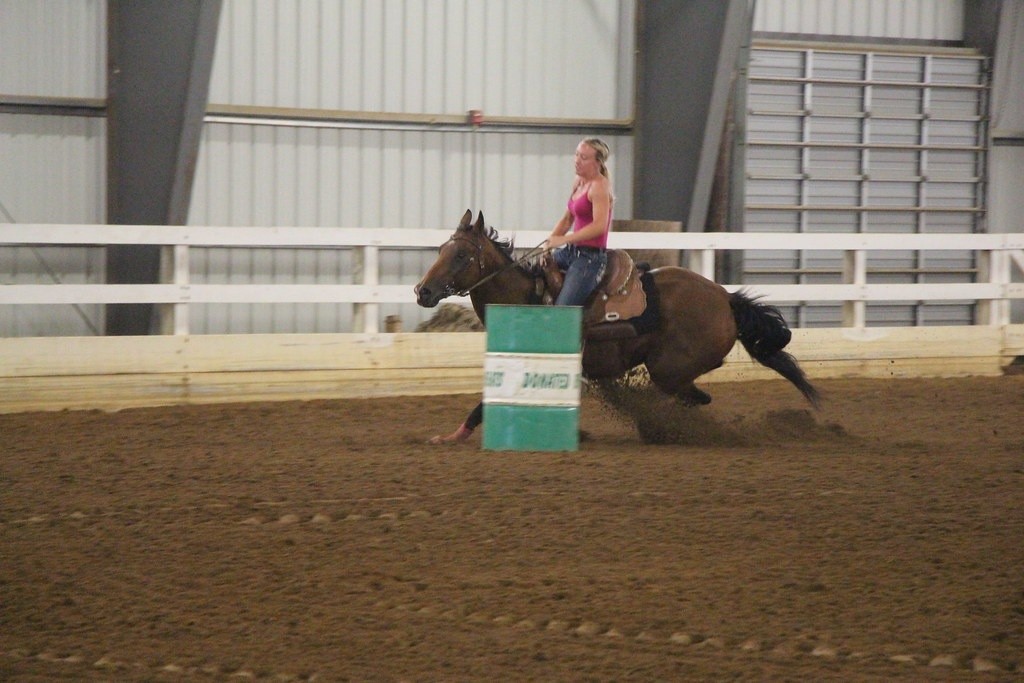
[544,136,613,304]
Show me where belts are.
[567,244,607,253]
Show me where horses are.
[413,209,823,443]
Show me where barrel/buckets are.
[484,303,585,452]
